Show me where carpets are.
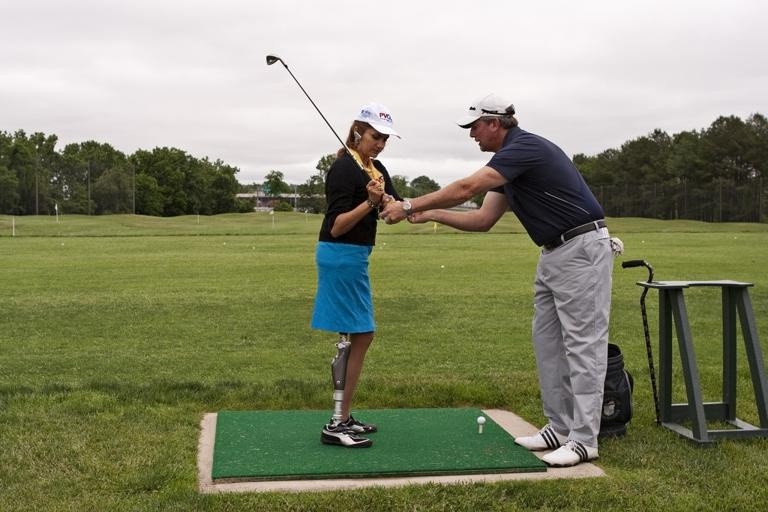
[212,408,547,478]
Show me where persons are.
[379,97,615,469]
[310,109,405,449]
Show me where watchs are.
[402,199,412,218]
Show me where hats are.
[450,92,516,128]
[354,103,402,139]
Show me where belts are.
[544,221,608,249]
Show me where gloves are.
[610,237,625,256]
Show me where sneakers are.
[320,414,377,448]
[541,440,600,467]
[514,424,568,451]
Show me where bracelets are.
[366,200,378,208]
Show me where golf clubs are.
[265,55,372,183]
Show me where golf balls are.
[476,416,485,422]
